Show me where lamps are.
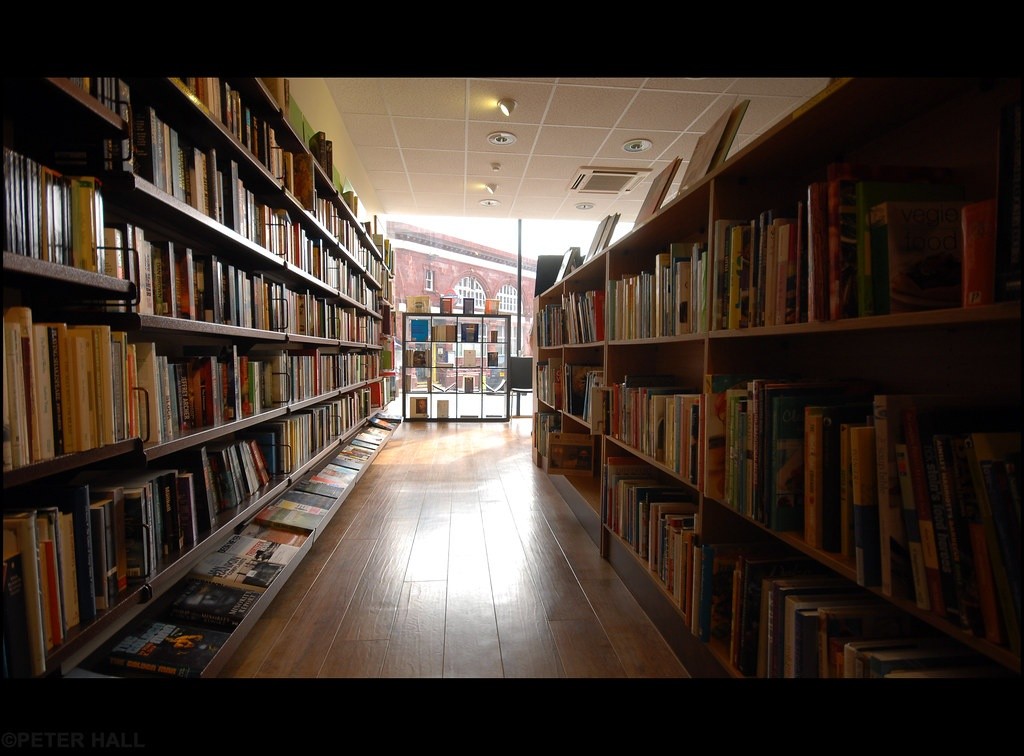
[486,184,497,193]
[497,97,519,116]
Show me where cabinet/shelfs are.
[530,77,1024,678]
[0,77,406,678]
[402,313,511,423]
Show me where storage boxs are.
[405,295,430,313]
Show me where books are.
[2,77,401,675]
[404,296,503,419]
[533,93,1018,679]
